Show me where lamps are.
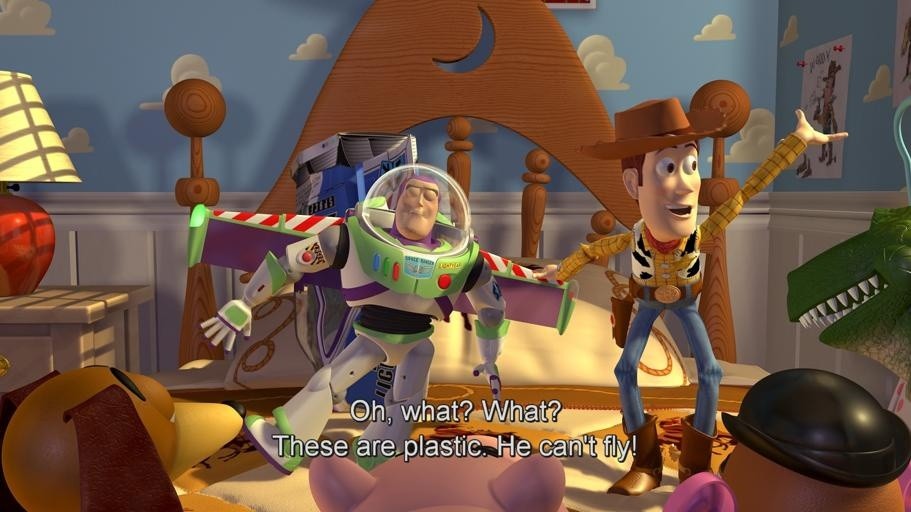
[0,68,83,300]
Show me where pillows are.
[224,256,686,390]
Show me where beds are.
[149,2,776,512]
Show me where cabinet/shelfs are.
[0,285,153,397]
